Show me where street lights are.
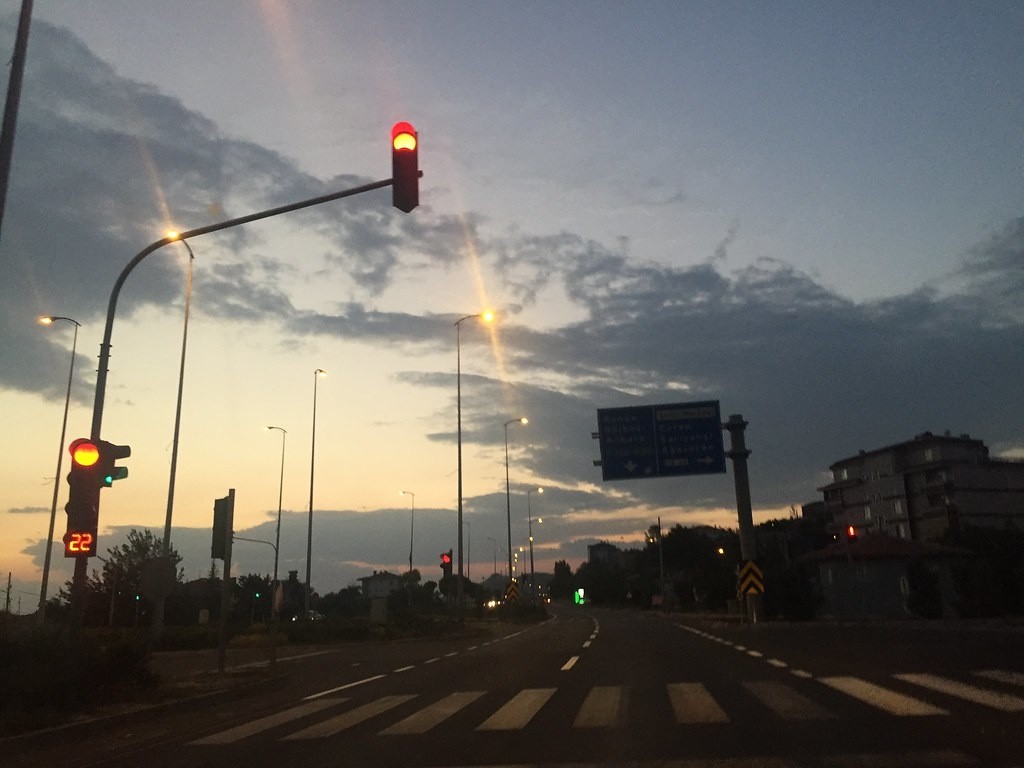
[37,316,83,628]
[505,417,528,584]
[268,426,287,619]
[526,487,544,588]
[402,491,414,611]
[453,309,496,602]
[303,368,327,625]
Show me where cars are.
[292,609,324,625]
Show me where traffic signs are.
[596,398,727,483]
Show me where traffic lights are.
[63,437,103,558]
[844,523,856,543]
[391,121,420,213]
[100,439,131,487]
[440,550,453,578]
